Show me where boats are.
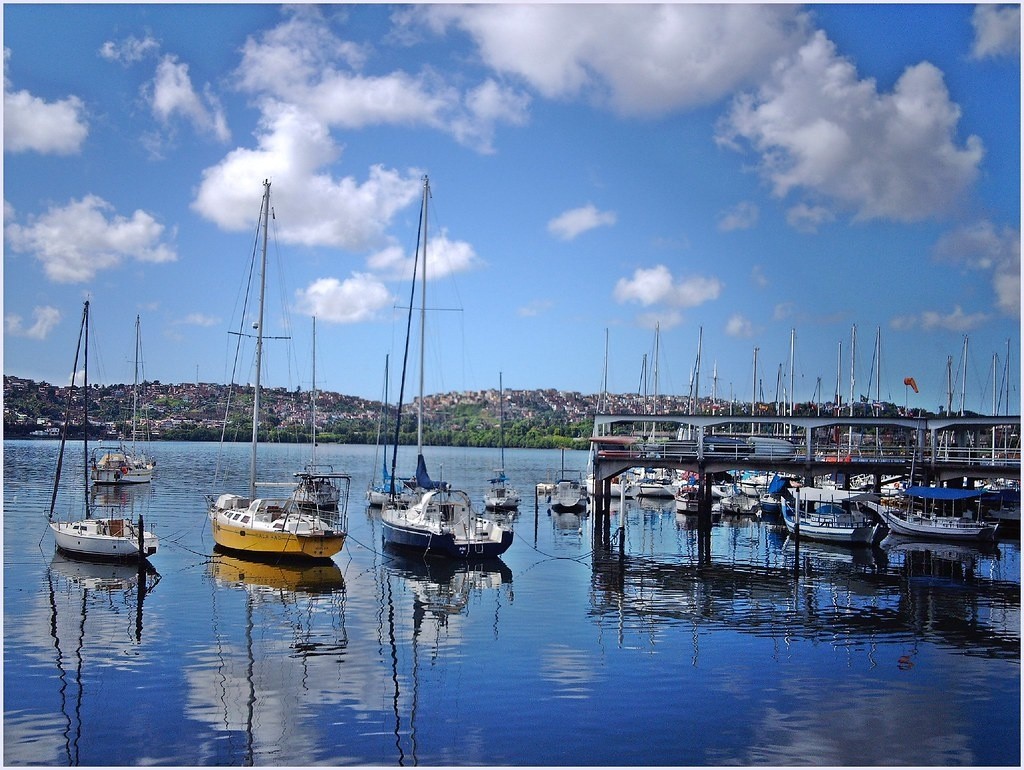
[781,494,890,543]
[865,497,999,540]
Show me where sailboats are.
[484,371,519,506]
[382,173,513,555]
[208,179,345,557]
[289,315,340,506]
[89,313,156,483]
[43,298,158,557]
[536,324,1021,515]
[366,354,433,506]
